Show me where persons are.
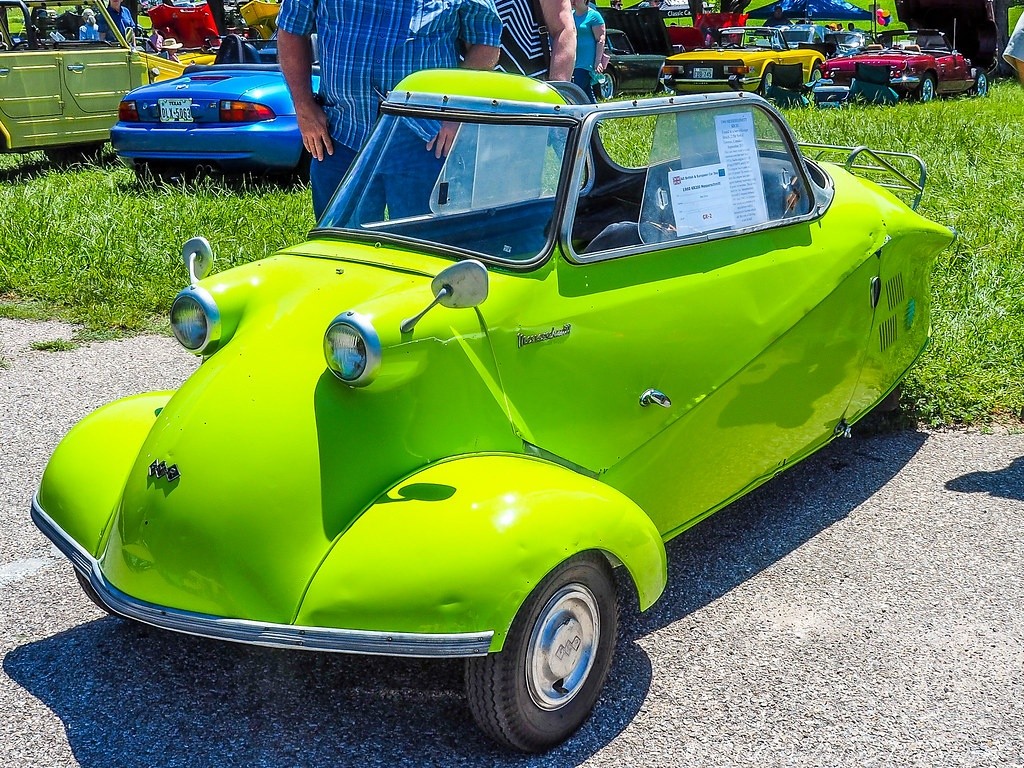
[647,1,662,8]
[273,0,608,227]
[31,0,183,64]
[762,6,793,27]
[824,23,857,32]
[609,1,624,12]
[1002,10,1024,80]
[795,18,814,24]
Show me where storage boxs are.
[813,87,850,108]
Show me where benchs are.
[761,158,812,219]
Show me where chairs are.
[585,220,662,253]
[849,62,899,104]
[868,44,883,51]
[310,35,319,65]
[770,63,816,108]
[905,45,921,52]
[214,34,261,64]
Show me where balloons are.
[877,8,891,27]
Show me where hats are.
[157,37,183,49]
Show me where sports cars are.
[664,26,826,99]
[109,33,320,188]
[813,16,989,109]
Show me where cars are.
[763,24,877,57]
[595,29,667,101]
[29,70,959,752]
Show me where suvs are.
[0,0,160,153]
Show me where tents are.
[746,0,873,51]
